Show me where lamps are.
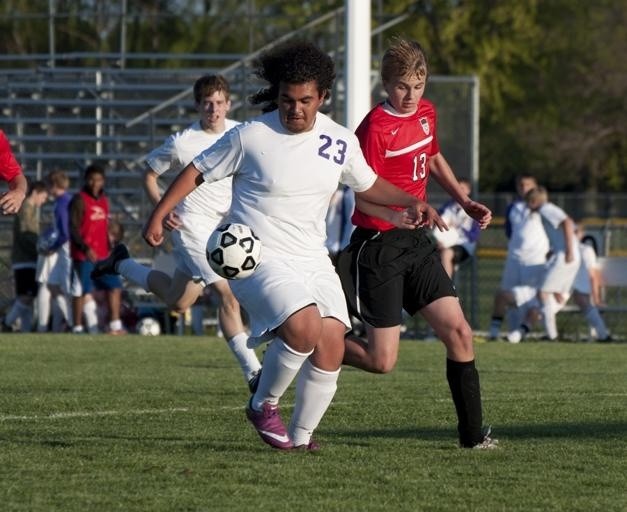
[560,255,627,342]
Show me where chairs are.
[245,393,294,450]
[459,423,504,454]
[90,243,130,281]
[248,361,264,396]
[295,441,321,452]
[486,325,616,343]
[1,314,128,336]
[560,255,627,342]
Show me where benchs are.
[0,50,372,336]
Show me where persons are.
[90,74,264,394]
[339,38,502,453]
[0,126,28,215]
[485,175,613,342]
[141,41,449,453]
[4,165,226,338]
[336,181,480,338]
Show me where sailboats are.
[207,223,262,281]
[136,318,160,336]
